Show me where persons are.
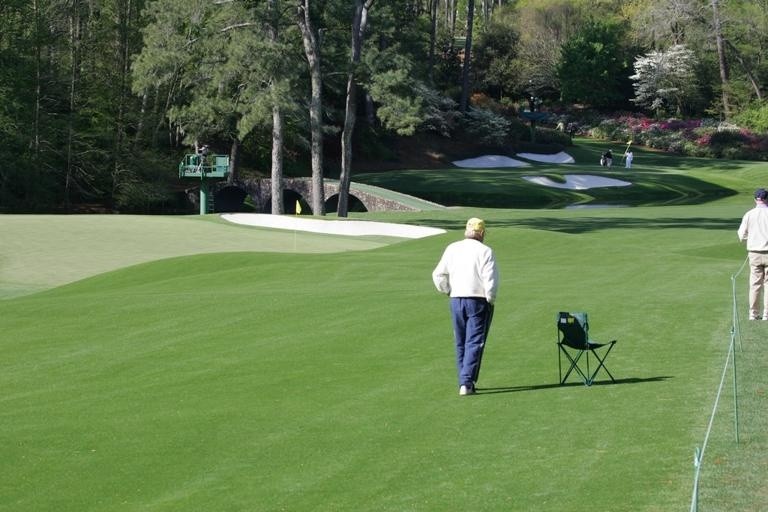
[197,143,211,172]
[431,217,499,397]
[605,148,613,169]
[735,187,768,322]
[600,154,606,166]
[554,120,564,133]
[623,148,633,169]
[566,122,577,138]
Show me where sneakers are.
[749,316,768,321]
[459,386,474,395]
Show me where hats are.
[754,189,768,201]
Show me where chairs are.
[557,311,617,387]
